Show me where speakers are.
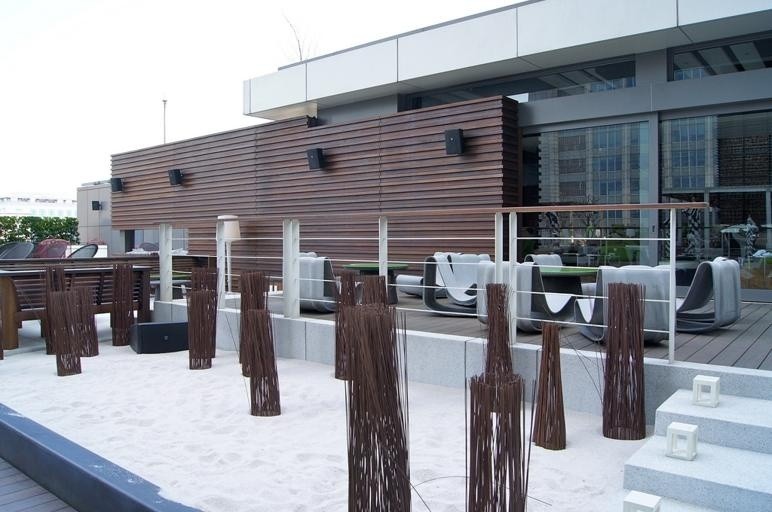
[167,169,181,185]
[306,147,323,170]
[444,129,465,156]
[92,201,99,211]
[111,178,122,191]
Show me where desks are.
[535,266,598,317]
[150,279,192,307]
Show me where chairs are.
[0,238,98,259]
[524,254,563,265]
[294,251,490,336]
[675,259,740,332]
[574,264,678,343]
[712,257,727,262]
[477,259,577,335]
